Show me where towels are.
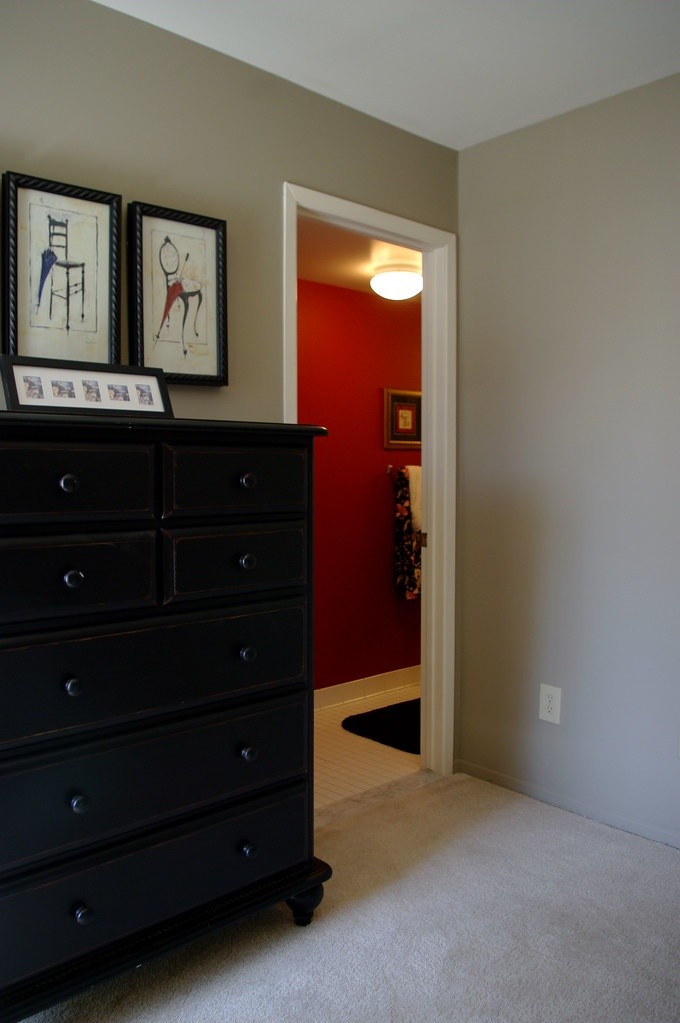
[405,465,422,531]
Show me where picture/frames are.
[2,360,173,418]
[1,171,121,372]
[127,201,228,387]
[383,389,421,449]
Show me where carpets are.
[342,697,420,756]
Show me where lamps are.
[370,264,423,301]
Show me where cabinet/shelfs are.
[0,408,332,1022]
